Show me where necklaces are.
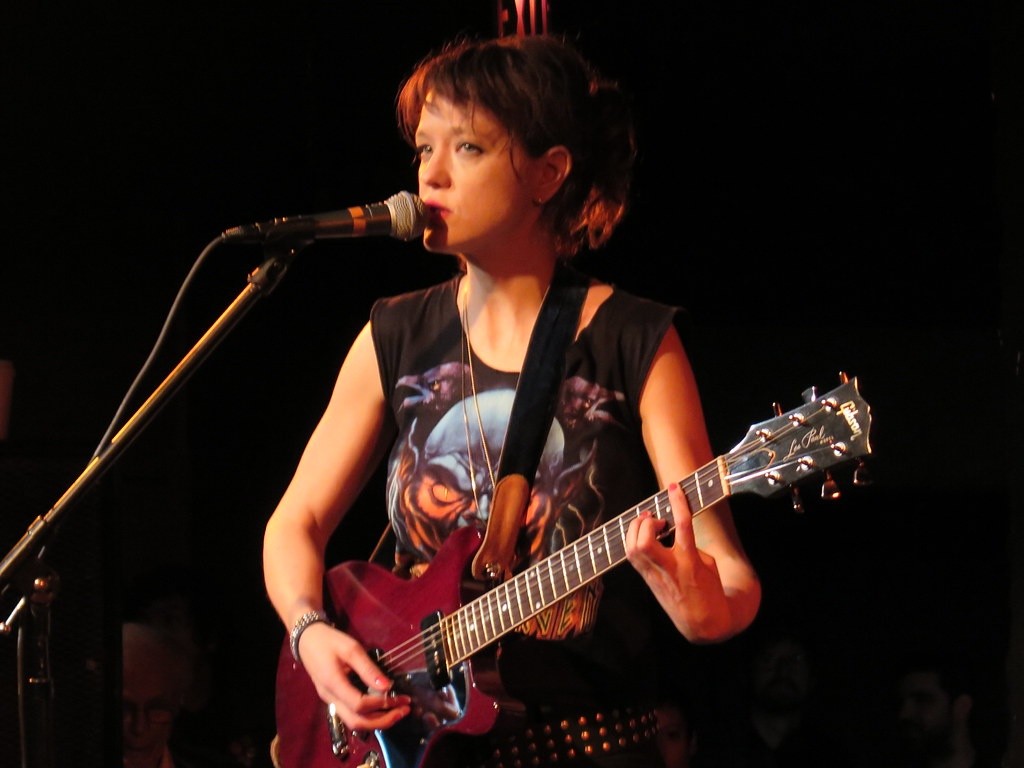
[462,284,495,523]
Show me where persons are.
[122,622,245,768]
[262,36,763,768]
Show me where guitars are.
[269,367,875,767]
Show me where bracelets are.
[289,611,331,662]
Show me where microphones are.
[221,191,430,243]
